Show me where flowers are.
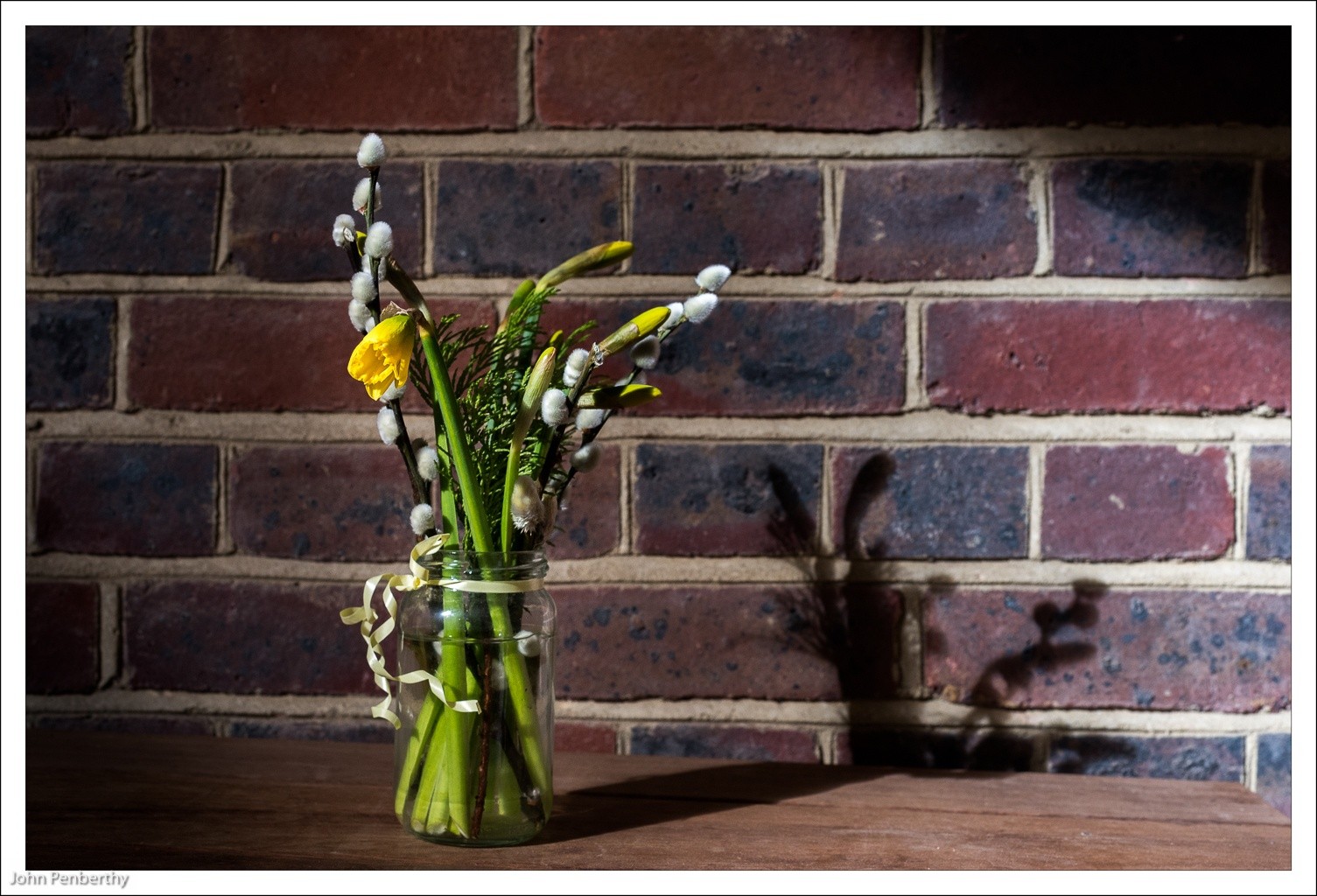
[330,129,730,838]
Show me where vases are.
[392,545,558,843]
